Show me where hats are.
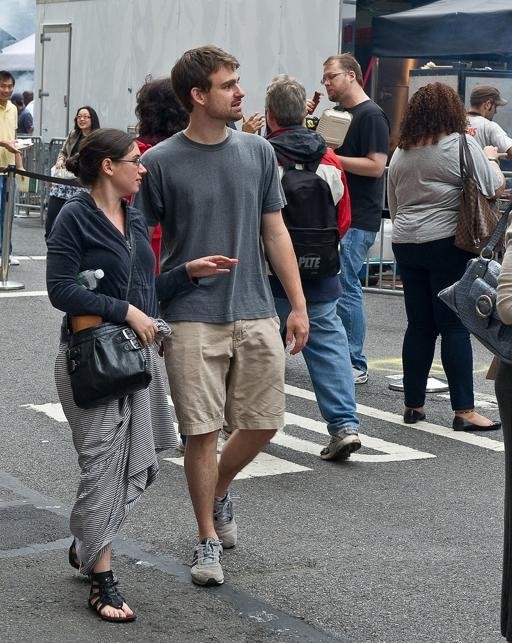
[469,85,508,107]
[11,93,25,102]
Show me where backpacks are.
[263,158,340,282]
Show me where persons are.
[131,46,310,586]
[387,82,508,431]
[23,90,34,118]
[486,203,512,643]
[11,94,34,135]
[463,84,512,161]
[304,54,389,385]
[46,127,239,623]
[0,71,26,267]
[133,79,265,280]
[45,107,101,242]
[257,74,362,462]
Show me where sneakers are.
[214,492,238,548]
[8,254,19,265]
[191,537,225,586]
[320,427,362,460]
[351,367,368,384]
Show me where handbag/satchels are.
[49,164,92,200]
[453,133,507,256]
[437,256,512,365]
[66,321,152,410]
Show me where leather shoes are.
[452,416,501,431]
[403,408,427,424]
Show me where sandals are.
[68,537,119,584]
[88,569,137,621]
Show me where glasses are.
[113,158,141,167]
[320,73,346,85]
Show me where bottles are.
[78,269,105,292]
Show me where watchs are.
[488,157,500,165]
